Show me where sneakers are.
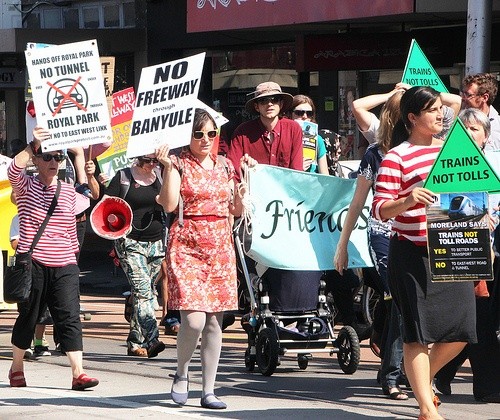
[33,345,51,356]
[25,348,33,357]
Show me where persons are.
[345,89,355,125]
[457,72,500,257]
[7,128,100,390]
[227,82,329,175]
[334,83,478,420]
[102,152,167,358]
[154,108,258,409]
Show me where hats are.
[245,81,293,116]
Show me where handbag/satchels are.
[3,251,33,304]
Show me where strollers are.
[234,217,361,374]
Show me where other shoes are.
[169,373,189,404]
[344,320,372,343]
[56,343,61,351]
[201,394,227,409]
[9,366,26,386]
[72,374,98,390]
[434,375,451,395]
[223,314,234,331]
[370,326,380,357]
[476,392,500,403]
[127,347,147,356]
[147,341,165,357]
[165,323,181,336]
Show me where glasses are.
[459,91,484,100]
[257,97,282,105]
[38,153,66,162]
[293,110,315,118]
[191,130,219,140]
[142,157,159,163]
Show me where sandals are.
[382,383,409,400]
[125,295,133,322]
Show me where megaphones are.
[90,196,133,239]
